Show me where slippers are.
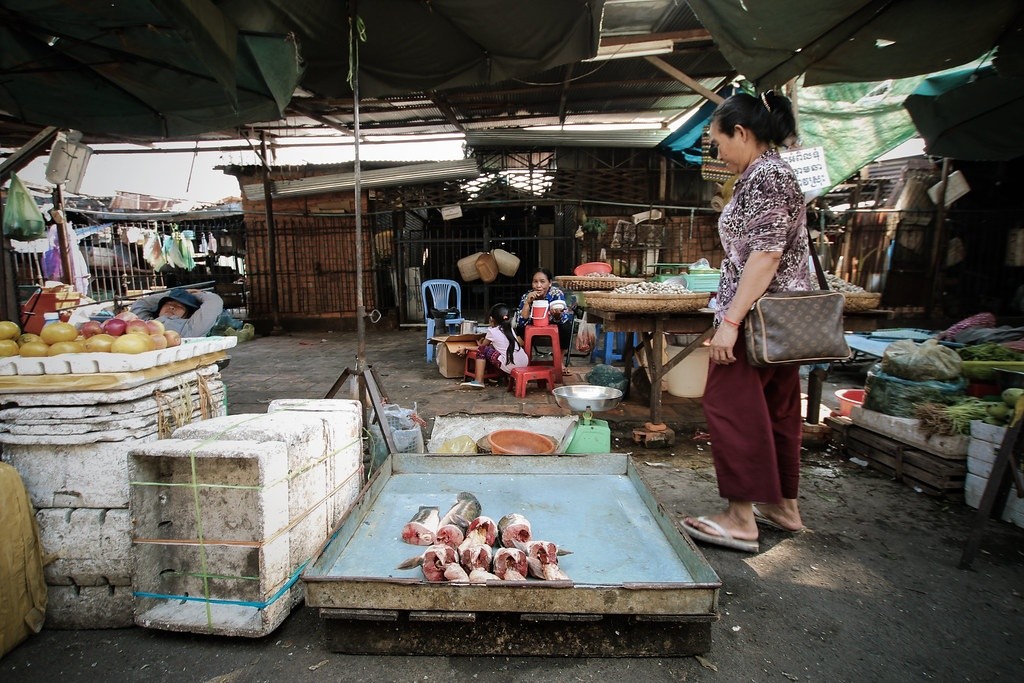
[460,380,485,389]
[680,515,760,553]
[753,503,802,533]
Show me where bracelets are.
[723,318,741,326]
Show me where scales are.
[647,263,692,288]
[552,384,624,454]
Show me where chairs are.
[422,279,466,362]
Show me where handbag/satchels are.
[746,289,852,371]
[2,171,48,242]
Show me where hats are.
[158,290,199,308]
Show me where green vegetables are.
[862,365,996,440]
[954,342,1024,362]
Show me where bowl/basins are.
[834,388,865,418]
[574,262,612,277]
[552,385,624,410]
[663,332,702,346]
[489,429,555,456]
[662,276,688,289]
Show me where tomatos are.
[0,311,181,359]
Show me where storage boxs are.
[426,333,488,378]
[966,421,1024,529]
[0,365,365,639]
[850,405,969,460]
[80,246,114,266]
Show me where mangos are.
[983,387,1024,427]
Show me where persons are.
[128,287,224,338]
[680,89,811,553]
[459,303,528,389]
[514,267,574,376]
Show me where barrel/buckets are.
[530,300,548,327]
[457,249,521,282]
[664,344,710,398]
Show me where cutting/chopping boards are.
[476,432,559,455]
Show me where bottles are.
[44,312,61,325]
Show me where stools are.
[524,324,563,384]
[507,366,554,398]
[590,322,638,368]
[463,351,506,386]
